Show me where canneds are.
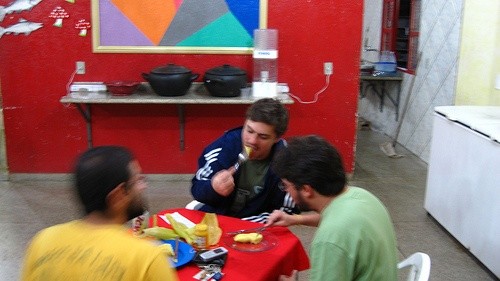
[193,225,209,251]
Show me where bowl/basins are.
[103,79,141,96]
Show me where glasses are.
[278,182,297,191]
[128,176,145,190]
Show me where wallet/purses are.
[195,250,226,266]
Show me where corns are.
[143,226,182,240]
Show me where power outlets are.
[323,62,334,75]
[76,61,86,74]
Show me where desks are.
[60,82,295,150]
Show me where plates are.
[223,229,278,252]
[161,239,196,267]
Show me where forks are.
[228,149,249,176]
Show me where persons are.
[263,135,398,281]
[189,98,302,223]
[20,145,177,281]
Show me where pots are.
[142,63,200,96]
[202,64,247,97]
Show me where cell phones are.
[199,246,228,262]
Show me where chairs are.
[396,252,432,281]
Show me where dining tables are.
[145,209,311,281]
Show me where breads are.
[232,233,263,244]
[245,146,253,156]
